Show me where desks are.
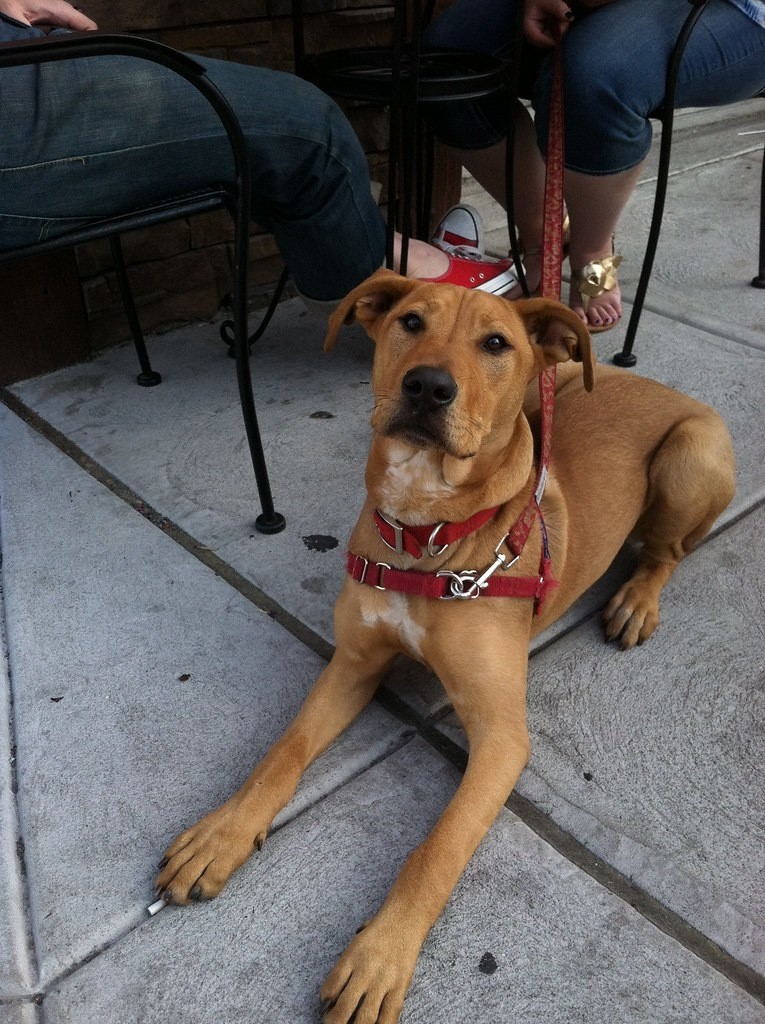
[515,1,765,368]
[227,0,532,359]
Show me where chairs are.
[0,30,286,536]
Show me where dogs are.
[149,269,733,1024]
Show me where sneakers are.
[414,247,525,297]
[429,204,500,263]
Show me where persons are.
[0,0,527,326]
[419,0,765,332]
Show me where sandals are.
[569,233,623,331]
[507,214,570,297]
